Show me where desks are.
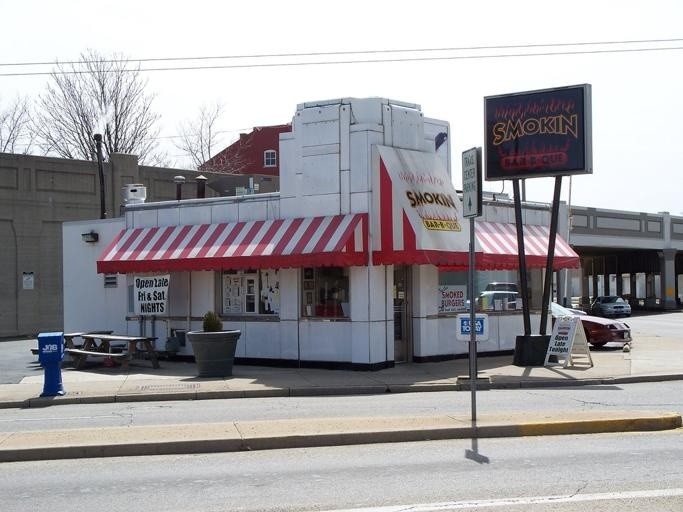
[63,330,113,348]
[73,334,158,372]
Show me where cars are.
[465,281,634,349]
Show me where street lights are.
[173,176,185,201]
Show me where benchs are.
[30,348,39,355]
[65,348,125,357]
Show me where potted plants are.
[186,312,241,377]
